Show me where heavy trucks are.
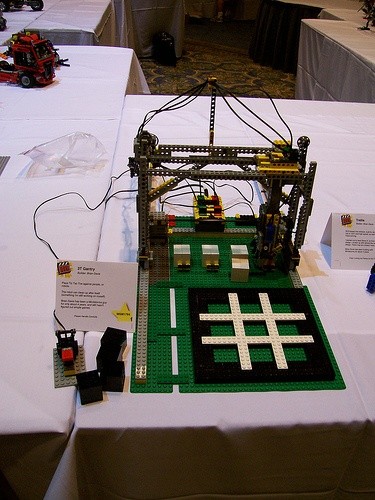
[0,33,56,87]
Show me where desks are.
[0,0,375,500]
